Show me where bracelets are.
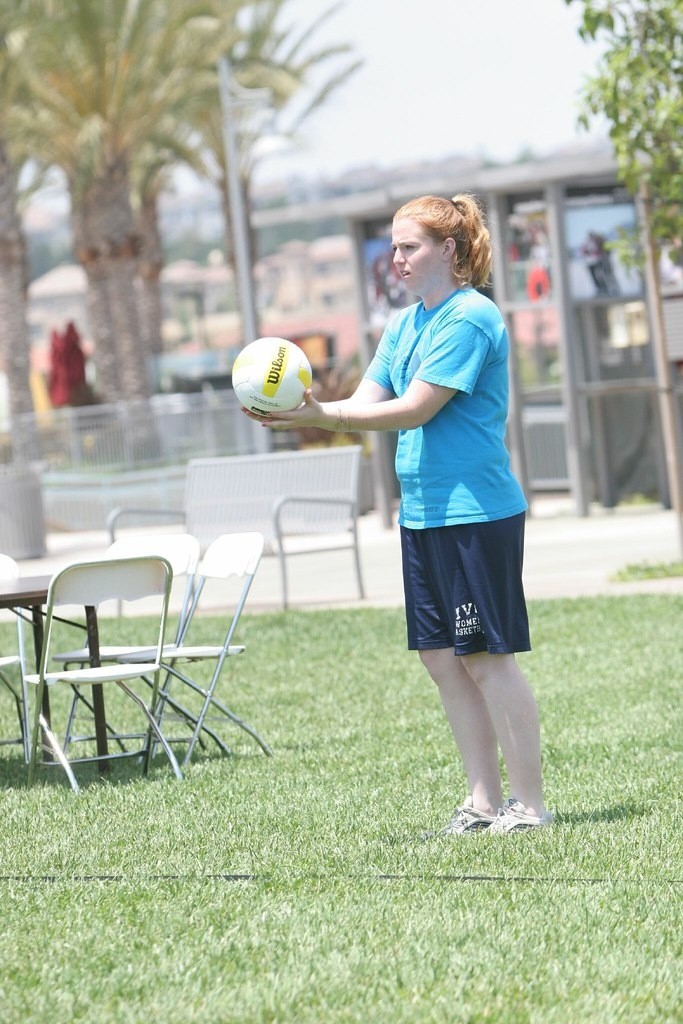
[337,407,349,433]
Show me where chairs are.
[0,530,272,780]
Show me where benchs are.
[108,446,365,614]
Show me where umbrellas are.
[46,324,84,466]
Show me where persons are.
[243,195,553,838]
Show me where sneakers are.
[443,795,496,837]
[480,797,552,836]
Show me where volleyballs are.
[231,333,313,421]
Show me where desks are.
[0,575,109,778]
